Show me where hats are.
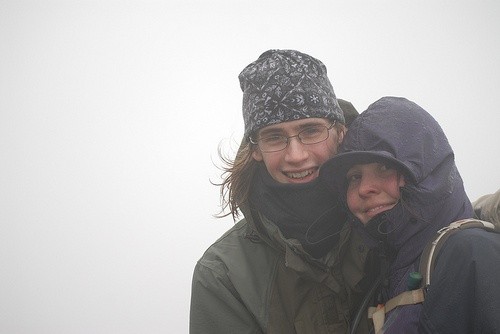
[237,48,345,139]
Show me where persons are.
[189,49,500,334]
[319,96,500,334]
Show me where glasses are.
[248,120,335,152]
